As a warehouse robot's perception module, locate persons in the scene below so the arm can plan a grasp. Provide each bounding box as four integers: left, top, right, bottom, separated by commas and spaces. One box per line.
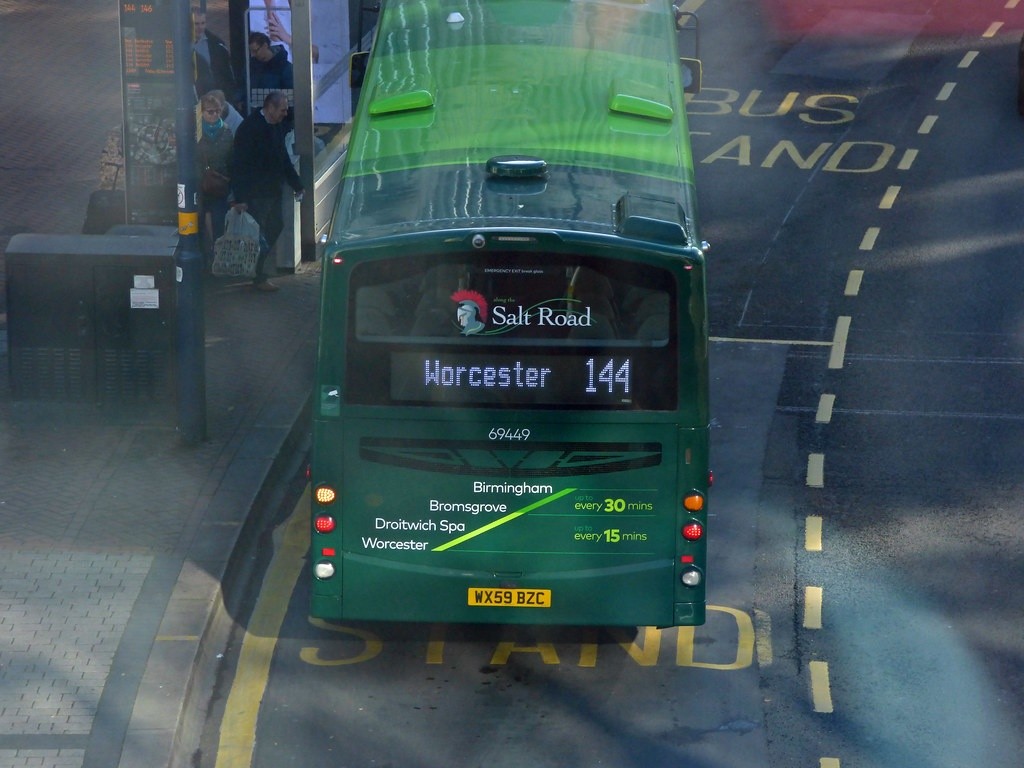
228, 92, 306, 291
249, 1, 319, 64
192, 7, 243, 259
249, 32, 295, 89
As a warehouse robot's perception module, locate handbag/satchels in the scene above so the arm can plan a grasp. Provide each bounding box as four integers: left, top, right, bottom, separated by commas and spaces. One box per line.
212, 207, 260, 279
203, 167, 232, 198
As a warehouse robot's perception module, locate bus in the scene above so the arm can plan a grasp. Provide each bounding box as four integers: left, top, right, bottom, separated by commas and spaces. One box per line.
304, 4, 711, 628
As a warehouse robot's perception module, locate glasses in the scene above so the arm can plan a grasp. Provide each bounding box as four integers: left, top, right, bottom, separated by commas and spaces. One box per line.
253, 45, 261, 53
204, 106, 223, 115
